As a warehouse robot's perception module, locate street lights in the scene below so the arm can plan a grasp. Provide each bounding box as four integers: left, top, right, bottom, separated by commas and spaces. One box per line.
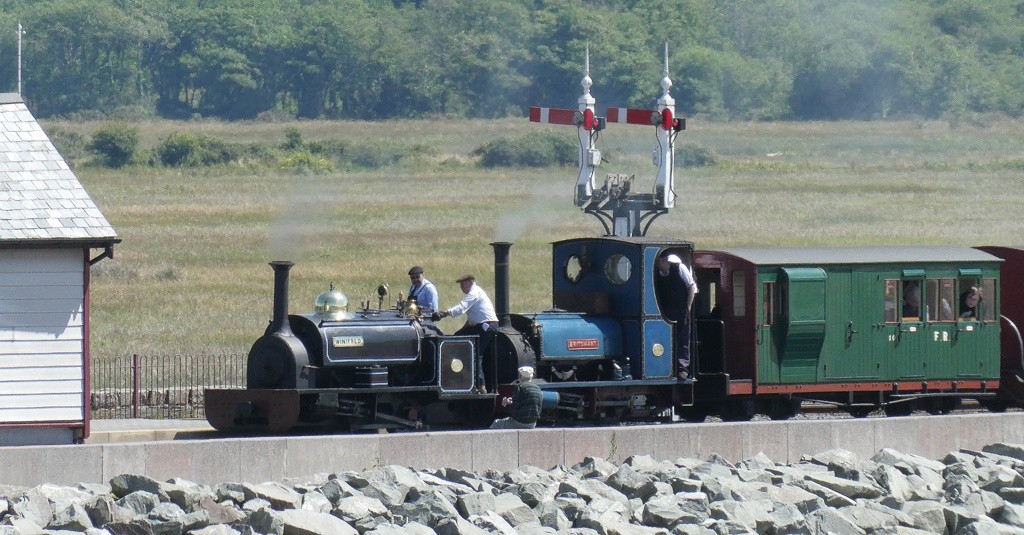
15, 20, 31, 94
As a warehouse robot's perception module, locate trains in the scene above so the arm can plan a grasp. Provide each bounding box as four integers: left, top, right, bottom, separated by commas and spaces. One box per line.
201, 40, 1024, 440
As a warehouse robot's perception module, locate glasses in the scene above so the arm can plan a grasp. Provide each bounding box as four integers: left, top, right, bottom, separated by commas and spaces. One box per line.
410, 275, 420, 279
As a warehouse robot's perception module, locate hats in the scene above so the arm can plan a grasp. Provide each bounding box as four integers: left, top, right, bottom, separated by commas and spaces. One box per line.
456, 274, 474, 282
408, 266, 423, 275
518, 366, 534, 379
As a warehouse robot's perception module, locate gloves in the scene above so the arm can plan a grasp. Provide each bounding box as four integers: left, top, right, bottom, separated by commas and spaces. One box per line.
431, 312, 445, 322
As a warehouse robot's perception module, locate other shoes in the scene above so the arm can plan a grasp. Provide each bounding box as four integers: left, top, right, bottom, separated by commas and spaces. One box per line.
678, 371, 688, 380
479, 386, 487, 394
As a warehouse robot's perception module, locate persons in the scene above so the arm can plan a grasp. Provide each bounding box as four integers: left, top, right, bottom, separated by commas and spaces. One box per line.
655, 250, 696, 379
960, 285, 983, 322
431, 275, 499, 394
407, 267, 438, 322
